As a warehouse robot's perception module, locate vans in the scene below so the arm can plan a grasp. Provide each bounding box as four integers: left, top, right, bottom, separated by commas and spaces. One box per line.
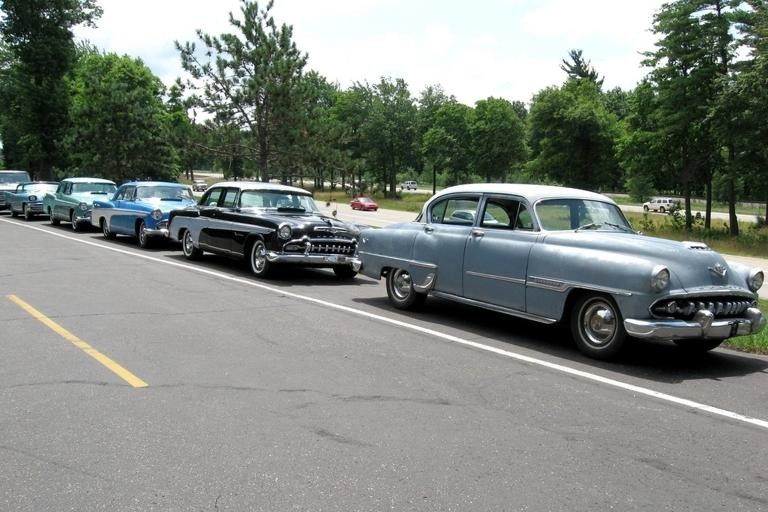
0, 170, 32, 210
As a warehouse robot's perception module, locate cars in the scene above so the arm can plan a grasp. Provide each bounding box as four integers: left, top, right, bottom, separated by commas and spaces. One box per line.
167, 181, 362, 280
350, 196, 378, 212
4, 181, 60, 221
91, 181, 197, 251
42, 177, 118, 232
351, 182, 765, 362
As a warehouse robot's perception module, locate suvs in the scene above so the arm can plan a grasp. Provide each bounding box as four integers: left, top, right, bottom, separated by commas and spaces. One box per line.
401, 180, 417, 190
192, 179, 208, 191
643, 196, 677, 213
